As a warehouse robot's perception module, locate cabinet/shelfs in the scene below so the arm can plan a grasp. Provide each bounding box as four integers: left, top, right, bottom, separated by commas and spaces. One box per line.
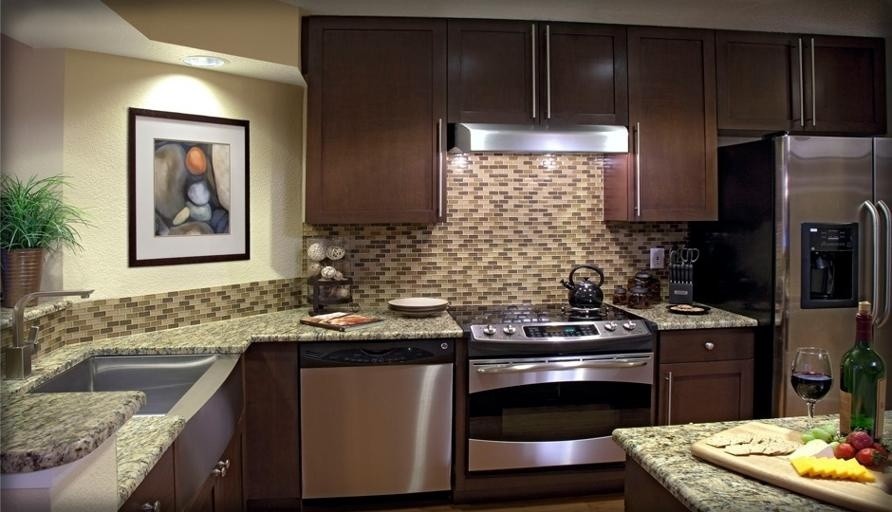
115, 442, 175, 509
714, 30, 890, 138
176, 359, 249, 512
601, 24, 716, 224
305, 12, 444, 226
659, 332, 754, 426
444, 17, 617, 123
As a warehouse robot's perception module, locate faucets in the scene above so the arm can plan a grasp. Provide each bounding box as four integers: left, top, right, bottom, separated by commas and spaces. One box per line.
5, 288, 96, 381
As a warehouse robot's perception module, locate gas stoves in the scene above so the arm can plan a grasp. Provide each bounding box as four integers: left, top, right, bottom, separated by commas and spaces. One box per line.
447, 302, 654, 357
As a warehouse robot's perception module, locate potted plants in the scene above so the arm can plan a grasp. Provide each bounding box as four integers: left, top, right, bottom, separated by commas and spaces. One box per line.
0, 170, 99, 307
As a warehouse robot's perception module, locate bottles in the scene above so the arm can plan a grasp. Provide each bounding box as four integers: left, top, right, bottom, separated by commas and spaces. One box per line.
836, 297, 884, 441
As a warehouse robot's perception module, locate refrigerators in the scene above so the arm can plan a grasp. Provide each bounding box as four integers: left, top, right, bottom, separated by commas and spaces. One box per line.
687, 135, 892, 418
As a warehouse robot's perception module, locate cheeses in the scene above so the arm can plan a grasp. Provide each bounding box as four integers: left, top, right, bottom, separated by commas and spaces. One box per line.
789, 456, 876, 482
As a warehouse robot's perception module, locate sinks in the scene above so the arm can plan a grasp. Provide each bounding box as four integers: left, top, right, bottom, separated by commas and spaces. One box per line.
10, 350, 246, 511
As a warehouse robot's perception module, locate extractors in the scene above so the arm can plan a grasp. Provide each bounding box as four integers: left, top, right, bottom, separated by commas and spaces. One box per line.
454, 122, 629, 158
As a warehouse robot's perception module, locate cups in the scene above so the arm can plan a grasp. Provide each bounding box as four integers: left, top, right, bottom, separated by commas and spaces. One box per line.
305, 237, 346, 281
612, 267, 661, 308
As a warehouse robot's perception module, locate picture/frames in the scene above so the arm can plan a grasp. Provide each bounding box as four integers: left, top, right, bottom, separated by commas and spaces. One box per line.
127, 106, 251, 267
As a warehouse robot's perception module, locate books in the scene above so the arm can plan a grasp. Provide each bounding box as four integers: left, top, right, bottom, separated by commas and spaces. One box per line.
300, 311, 385, 332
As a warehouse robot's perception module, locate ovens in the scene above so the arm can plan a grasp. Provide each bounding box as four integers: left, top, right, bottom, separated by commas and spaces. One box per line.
467, 351, 657, 477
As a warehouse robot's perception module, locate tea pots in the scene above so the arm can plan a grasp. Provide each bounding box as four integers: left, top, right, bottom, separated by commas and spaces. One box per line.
558, 264, 603, 308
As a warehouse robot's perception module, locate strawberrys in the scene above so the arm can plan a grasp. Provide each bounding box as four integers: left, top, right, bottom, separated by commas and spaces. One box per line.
835, 431, 891, 467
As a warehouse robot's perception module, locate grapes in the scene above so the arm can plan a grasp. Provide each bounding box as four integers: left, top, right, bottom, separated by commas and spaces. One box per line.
800, 425, 838, 444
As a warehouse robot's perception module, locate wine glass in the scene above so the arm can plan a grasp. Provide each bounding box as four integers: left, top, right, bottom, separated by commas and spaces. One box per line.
788, 343, 836, 432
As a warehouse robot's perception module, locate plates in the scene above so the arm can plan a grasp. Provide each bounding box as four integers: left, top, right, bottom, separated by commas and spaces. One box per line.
665, 304, 711, 314
386, 297, 448, 318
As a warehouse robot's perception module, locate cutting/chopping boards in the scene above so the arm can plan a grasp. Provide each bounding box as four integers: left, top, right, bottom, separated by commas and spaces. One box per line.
691, 419, 892, 512
